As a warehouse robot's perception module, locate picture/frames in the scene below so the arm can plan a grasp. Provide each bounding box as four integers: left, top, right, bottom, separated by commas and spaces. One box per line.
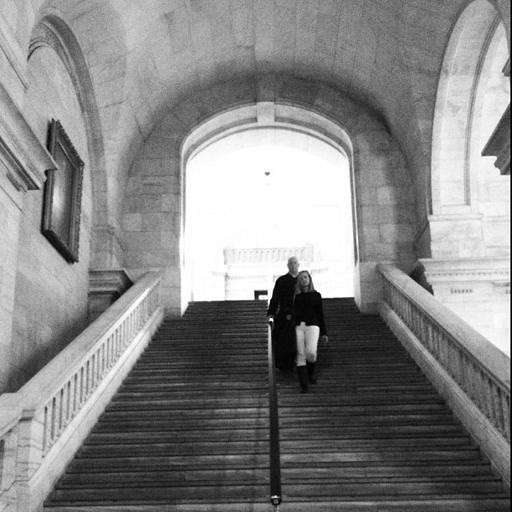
41, 118, 84, 264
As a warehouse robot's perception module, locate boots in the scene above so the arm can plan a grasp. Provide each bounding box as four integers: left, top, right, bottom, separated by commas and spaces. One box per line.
298, 365, 309, 394
307, 360, 317, 383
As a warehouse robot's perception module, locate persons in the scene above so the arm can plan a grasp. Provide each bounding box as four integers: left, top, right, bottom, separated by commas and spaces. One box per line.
266, 256, 301, 385
285, 270, 329, 394
